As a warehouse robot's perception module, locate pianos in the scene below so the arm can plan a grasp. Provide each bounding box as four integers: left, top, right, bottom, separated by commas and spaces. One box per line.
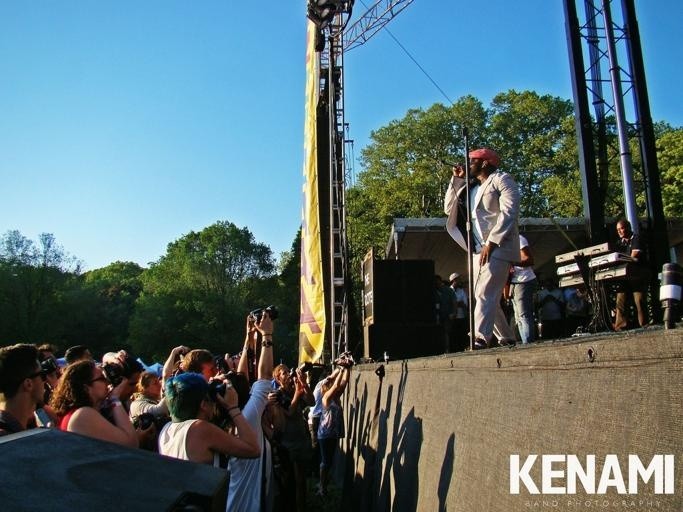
555, 242, 654, 289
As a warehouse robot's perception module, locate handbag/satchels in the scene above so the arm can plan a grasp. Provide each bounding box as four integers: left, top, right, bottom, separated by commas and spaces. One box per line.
273, 430, 300, 468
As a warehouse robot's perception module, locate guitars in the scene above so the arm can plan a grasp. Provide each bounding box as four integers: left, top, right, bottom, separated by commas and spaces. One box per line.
503, 265, 514, 301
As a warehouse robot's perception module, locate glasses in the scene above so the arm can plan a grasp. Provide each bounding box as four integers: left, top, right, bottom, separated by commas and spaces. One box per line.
33, 367, 48, 384
469, 159, 478, 165
88, 371, 108, 387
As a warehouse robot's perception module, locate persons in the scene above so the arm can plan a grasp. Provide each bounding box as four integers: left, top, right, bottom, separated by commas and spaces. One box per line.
0, 308, 356, 510
444, 148, 522, 349
431, 271, 596, 353
611, 219, 647, 330
507, 232, 539, 344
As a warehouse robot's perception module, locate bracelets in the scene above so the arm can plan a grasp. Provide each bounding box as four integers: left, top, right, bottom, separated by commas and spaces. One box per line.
483, 240, 496, 248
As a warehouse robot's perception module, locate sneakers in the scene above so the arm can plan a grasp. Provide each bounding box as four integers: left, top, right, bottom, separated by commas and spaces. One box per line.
318, 489, 329, 505
316, 483, 329, 495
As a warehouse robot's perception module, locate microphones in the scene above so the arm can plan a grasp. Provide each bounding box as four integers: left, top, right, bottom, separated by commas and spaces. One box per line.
442, 160, 465, 170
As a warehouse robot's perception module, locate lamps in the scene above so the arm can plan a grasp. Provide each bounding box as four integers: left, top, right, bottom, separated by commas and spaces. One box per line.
306, 0, 331, 30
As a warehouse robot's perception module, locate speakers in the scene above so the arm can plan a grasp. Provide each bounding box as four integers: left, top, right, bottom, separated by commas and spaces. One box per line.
370, 259, 435, 362
0, 427, 230, 512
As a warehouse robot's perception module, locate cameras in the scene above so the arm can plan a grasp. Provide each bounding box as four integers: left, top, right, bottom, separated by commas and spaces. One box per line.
40, 357, 56, 375
250, 305, 278, 322
209, 381, 225, 402
105, 360, 129, 385
289, 361, 313, 378
139, 413, 153, 430
216, 357, 229, 374
271, 389, 291, 412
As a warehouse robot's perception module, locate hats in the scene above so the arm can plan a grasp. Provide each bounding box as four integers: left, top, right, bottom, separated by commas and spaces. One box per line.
448, 272, 460, 282
467, 147, 501, 169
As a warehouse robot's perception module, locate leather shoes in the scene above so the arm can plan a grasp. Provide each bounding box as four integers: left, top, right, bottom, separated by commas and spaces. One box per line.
472, 338, 487, 350
611, 320, 627, 331
